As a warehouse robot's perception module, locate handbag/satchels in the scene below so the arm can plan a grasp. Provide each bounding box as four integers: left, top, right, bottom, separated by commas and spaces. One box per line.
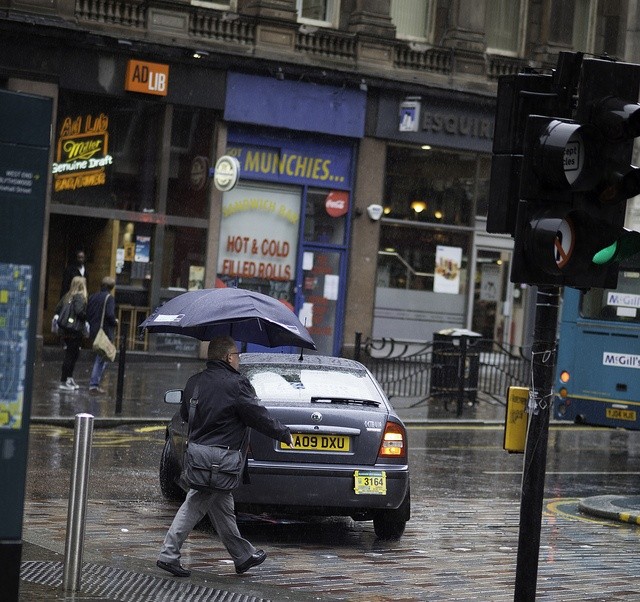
179, 370, 252, 492
92, 293, 117, 363
57, 294, 90, 340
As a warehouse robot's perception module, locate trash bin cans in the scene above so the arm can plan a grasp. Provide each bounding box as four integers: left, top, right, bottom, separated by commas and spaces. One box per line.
429, 328, 482, 419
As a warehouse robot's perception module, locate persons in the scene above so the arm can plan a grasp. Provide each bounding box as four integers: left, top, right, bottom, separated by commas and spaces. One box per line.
60, 249, 89, 303
155, 335, 296, 577
59, 274, 89, 395
87, 275, 116, 395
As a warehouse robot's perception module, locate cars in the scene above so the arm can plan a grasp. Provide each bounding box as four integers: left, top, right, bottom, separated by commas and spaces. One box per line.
159, 320, 410, 542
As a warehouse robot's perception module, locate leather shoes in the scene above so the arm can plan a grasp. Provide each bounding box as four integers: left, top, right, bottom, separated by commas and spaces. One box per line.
235, 549, 267, 574
156, 559, 191, 577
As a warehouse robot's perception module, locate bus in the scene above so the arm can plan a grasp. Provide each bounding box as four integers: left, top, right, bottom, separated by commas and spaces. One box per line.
555, 273, 640, 431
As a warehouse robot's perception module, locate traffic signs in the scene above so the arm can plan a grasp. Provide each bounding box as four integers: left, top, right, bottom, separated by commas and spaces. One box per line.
511, 113, 592, 285
486, 73, 557, 232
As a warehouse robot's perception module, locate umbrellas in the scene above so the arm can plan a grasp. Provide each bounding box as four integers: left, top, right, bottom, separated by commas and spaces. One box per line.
137, 288, 319, 353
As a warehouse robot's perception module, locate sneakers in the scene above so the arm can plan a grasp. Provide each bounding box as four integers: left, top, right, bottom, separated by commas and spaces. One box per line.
89, 385, 104, 393
59, 380, 74, 391
65, 377, 80, 390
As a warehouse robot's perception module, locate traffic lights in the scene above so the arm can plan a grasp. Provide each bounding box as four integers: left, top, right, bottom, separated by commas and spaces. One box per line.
564, 57, 640, 287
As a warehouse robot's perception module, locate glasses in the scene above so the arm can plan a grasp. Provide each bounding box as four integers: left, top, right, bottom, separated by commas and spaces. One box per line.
230, 351, 243, 357
